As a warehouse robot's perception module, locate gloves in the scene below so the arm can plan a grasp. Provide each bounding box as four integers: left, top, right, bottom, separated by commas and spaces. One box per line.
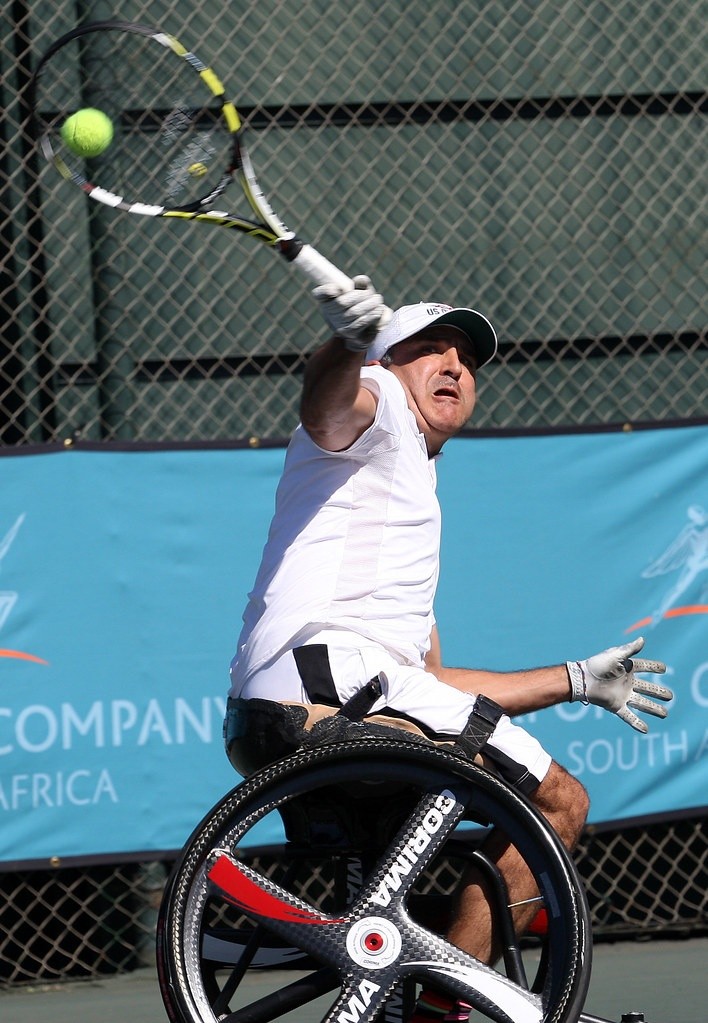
567, 637, 673, 733
312, 275, 395, 353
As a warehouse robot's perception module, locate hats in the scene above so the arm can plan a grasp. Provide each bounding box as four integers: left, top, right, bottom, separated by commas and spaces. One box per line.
365, 301, 497, 373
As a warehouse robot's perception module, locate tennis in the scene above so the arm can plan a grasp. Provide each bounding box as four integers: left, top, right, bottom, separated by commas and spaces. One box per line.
61, 108, 118, 157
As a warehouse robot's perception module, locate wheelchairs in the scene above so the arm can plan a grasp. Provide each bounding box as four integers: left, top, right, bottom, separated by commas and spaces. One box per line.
155, 673, 648, 1023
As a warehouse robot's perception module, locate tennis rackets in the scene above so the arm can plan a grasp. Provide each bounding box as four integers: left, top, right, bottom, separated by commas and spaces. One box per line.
30, 19, 395, 333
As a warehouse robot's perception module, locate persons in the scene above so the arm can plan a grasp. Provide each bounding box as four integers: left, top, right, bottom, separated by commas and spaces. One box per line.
225, 275, 673, 1021
